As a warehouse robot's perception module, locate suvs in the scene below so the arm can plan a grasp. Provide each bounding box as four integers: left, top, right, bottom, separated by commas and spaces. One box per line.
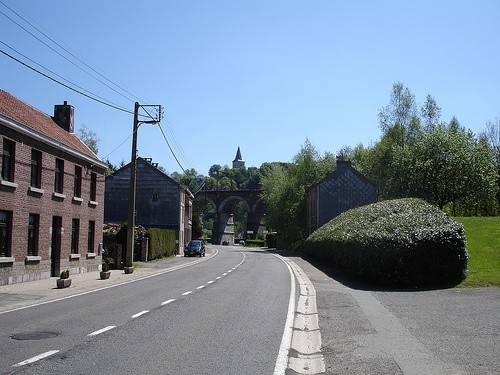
184, 240, 205, 258
